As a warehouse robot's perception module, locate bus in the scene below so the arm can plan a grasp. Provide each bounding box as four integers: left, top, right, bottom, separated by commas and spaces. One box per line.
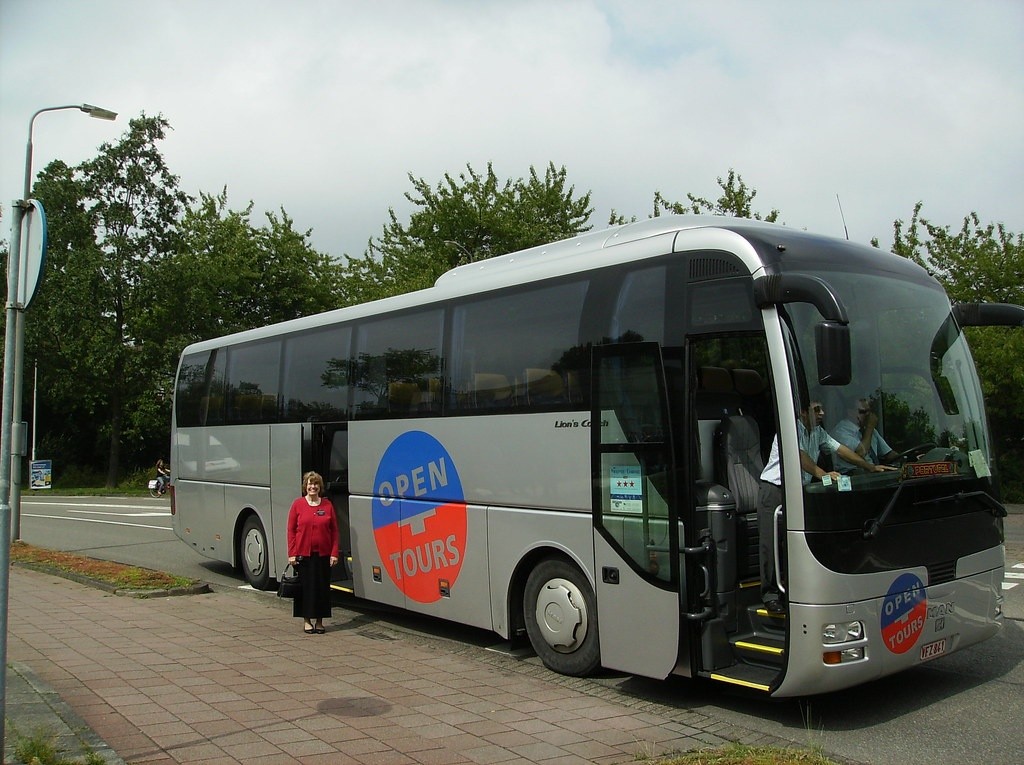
168, 212, 1024, 701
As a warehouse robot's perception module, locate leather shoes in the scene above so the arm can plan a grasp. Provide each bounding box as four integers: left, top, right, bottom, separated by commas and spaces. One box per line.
304, 624, 315, 634
760, 596, 784, 613
315, 624, 325, 634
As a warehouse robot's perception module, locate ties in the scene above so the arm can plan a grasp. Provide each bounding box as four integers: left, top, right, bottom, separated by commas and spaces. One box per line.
859, 427, 879, 464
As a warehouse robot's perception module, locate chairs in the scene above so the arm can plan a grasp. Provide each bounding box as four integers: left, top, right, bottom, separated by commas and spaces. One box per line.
386, 365, 586, 413
698, 364, 729, 407
715, 413, 770, 518
200, 389, 316, 422
733, 362, 779, 402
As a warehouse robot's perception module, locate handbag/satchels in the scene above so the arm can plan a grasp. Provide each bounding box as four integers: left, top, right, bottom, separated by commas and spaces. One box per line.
276, 562, 305, 598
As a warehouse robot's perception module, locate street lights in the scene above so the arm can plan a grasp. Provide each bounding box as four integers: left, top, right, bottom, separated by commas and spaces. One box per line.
0, 103, 118, 765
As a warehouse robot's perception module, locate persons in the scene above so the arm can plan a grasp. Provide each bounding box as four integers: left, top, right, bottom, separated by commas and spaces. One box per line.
759, 393, 897, 613
831, 396, 924, 476
157, 459, 170, 495
287, 472, 339, 634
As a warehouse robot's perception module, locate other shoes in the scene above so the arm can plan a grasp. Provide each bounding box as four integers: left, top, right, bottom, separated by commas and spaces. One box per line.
157, 491, 161, 495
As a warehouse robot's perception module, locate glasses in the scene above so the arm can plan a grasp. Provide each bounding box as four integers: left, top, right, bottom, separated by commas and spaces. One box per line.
859, 409, 867, 414
808, 405, 820, 412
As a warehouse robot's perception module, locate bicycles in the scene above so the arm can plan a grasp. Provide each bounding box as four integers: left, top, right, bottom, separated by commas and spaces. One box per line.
149, 471, 170, 498
